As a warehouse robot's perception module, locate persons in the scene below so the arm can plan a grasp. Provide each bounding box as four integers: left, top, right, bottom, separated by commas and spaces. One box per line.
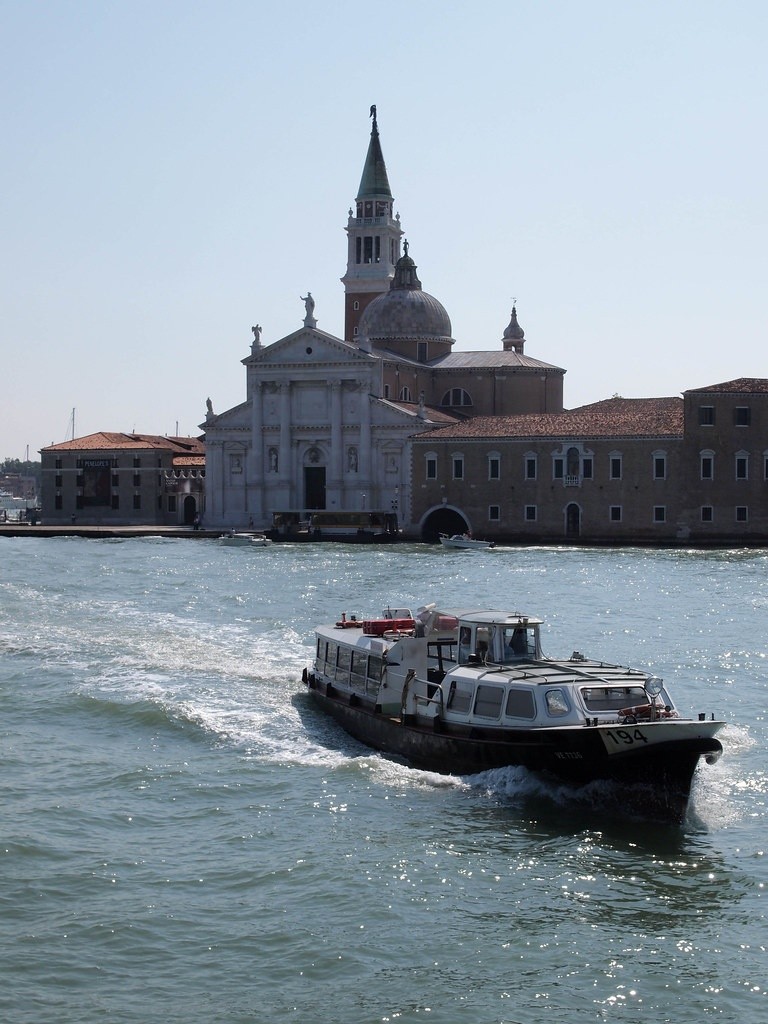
464, 530, 471, 541
300, 292, 315, 320
270, 451, 277, 471
193, 512, 200, 530
71, 514, 75, 525
403, 239, 409, 255
307, 518, 311, 533
417, 391, 425, 409
248, 517, 254, 530
252, 324, 262, 342
368, 513, 374, 528
349, 451, 357, 469
228, 528, 235, 538
206, 397, 212, 411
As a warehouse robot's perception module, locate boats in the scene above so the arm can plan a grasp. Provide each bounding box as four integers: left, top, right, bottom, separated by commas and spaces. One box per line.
438, 532, 495, 549
301, 602, 728, 827
268, 507, 399, 544
217, 529, 273, 547
